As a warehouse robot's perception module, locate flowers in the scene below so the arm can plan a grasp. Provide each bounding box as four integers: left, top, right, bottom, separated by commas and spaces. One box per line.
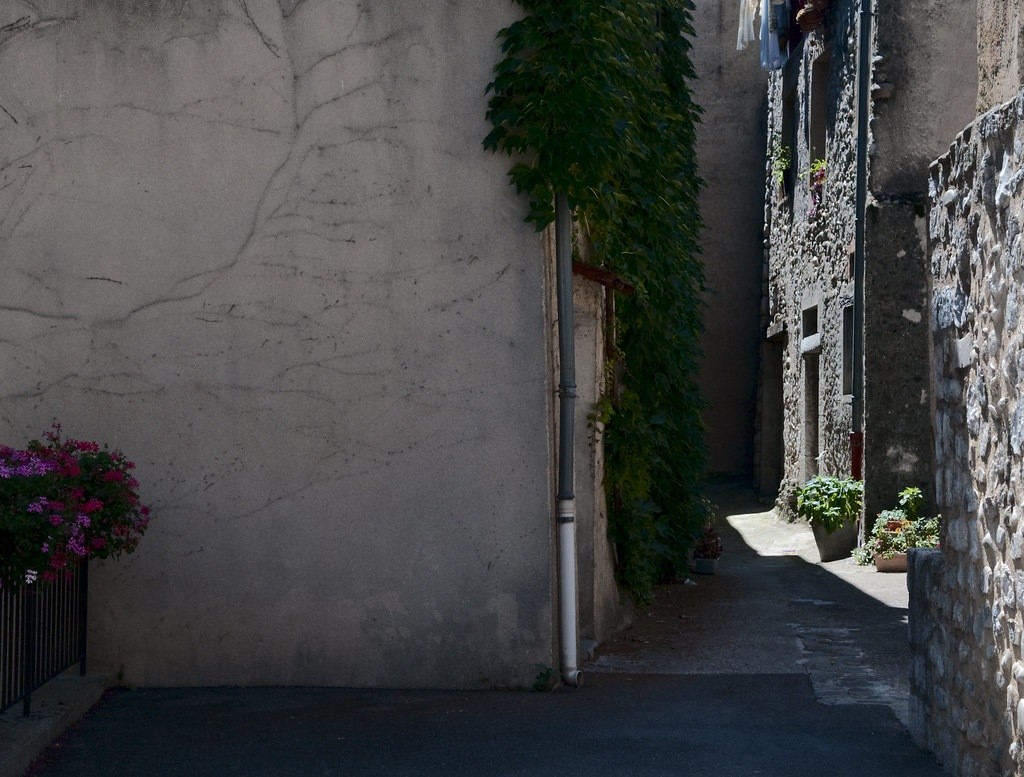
0, 420, 155, 584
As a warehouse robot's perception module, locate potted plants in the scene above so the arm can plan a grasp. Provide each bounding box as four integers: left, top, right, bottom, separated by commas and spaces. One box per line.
800, 159, 826, 203
866, 486, 942, 572
692, 529, 723, 575
796, 474, 864, 562
